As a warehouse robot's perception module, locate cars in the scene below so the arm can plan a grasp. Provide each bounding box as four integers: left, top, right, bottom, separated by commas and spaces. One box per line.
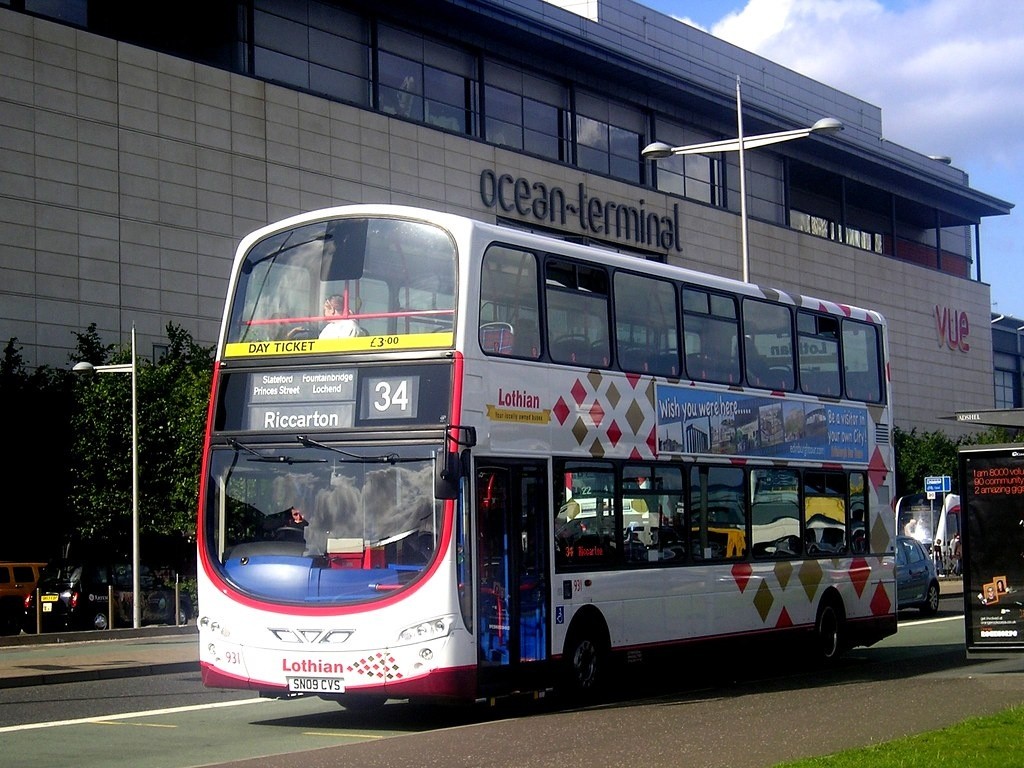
21, 554, 192, 631
896, 535, 940, 616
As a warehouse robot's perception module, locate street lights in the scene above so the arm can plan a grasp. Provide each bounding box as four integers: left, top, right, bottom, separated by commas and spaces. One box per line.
70, 321, 144, 628
636, 73, 844, 282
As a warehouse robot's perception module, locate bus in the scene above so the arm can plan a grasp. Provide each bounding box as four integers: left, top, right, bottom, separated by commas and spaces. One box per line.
194, 202, 900, 719
895, 492, 960, 576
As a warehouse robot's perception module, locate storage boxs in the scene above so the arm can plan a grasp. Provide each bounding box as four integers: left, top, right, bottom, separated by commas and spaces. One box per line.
327, 538, 386, 570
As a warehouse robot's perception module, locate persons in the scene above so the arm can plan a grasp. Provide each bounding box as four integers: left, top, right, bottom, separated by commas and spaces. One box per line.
932, 533, 962, 577
313, 295, 368, 337
280, 505, 309, 543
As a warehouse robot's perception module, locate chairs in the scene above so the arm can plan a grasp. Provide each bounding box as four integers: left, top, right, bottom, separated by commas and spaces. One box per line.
479, 321, 873, 400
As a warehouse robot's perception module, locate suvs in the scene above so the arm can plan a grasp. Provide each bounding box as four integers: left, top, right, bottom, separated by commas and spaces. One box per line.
0, 561, 50, 628
